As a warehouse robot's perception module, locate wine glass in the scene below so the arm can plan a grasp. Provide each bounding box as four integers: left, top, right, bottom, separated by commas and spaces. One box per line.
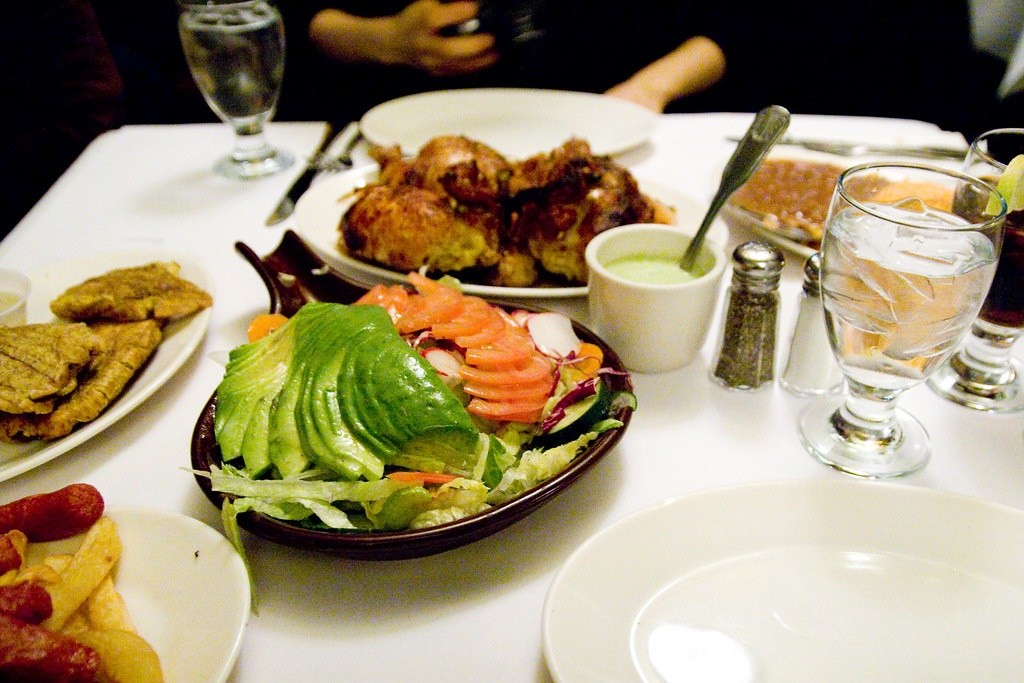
797, 162, 1007, 477
925, 127, 1024, 413
177, 2, 296, 180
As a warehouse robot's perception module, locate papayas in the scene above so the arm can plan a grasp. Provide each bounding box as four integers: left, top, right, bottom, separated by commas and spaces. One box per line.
216, 303, 479, 480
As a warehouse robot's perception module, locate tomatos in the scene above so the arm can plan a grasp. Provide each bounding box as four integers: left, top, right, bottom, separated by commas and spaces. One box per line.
352, 272, 552, 423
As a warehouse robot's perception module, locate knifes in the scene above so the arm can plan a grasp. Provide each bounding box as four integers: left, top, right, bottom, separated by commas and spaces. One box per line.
266, 121, 350, 225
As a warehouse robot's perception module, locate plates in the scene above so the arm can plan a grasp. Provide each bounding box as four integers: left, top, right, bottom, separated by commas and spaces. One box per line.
191, 230, 636, 560
0, 249, 213, 482
541, 478, 1024, 683
360, 88, 660, 156
714, 147, 854, 241
289, 158, 701, 297
27, 505, 252, 683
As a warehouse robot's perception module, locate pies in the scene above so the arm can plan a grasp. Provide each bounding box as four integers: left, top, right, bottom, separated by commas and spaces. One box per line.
0, 261, 212, 442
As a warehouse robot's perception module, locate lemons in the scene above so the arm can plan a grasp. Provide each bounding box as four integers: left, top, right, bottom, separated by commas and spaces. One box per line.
985, 154, 1024, 215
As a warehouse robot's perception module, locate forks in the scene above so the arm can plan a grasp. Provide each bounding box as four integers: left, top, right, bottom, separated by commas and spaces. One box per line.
303, 130, 362, 172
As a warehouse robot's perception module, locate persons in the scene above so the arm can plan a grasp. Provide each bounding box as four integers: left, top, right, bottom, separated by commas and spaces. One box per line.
284, 0, 776, 132
0, 0, 125, 225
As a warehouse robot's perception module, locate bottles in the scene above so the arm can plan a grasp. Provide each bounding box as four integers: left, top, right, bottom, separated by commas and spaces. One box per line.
710, 240, 786, 392
778, 252, 845, 399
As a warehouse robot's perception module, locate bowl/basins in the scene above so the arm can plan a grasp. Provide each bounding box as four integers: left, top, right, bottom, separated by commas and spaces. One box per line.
0, 268, 32, 328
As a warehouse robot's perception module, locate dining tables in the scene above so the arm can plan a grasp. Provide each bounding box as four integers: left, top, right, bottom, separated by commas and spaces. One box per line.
0, 116, 1024, 683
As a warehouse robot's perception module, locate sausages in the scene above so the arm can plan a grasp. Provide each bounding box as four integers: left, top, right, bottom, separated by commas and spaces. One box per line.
0, 483, 116, 683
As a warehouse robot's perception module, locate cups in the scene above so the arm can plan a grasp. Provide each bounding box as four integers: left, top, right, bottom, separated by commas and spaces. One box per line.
585, 223, 725, 373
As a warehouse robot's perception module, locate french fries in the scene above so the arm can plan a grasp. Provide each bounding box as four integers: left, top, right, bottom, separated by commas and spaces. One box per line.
0, 515, 163, 683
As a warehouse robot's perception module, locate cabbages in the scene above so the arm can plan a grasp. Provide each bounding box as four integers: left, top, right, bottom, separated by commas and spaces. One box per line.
181, 423, 596, 615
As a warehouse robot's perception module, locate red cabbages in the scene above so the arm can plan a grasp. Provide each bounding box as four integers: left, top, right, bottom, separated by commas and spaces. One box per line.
402, 304, 630, 433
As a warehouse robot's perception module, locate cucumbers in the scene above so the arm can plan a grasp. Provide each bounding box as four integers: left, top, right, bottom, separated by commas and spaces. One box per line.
538, 381, 610, 444
